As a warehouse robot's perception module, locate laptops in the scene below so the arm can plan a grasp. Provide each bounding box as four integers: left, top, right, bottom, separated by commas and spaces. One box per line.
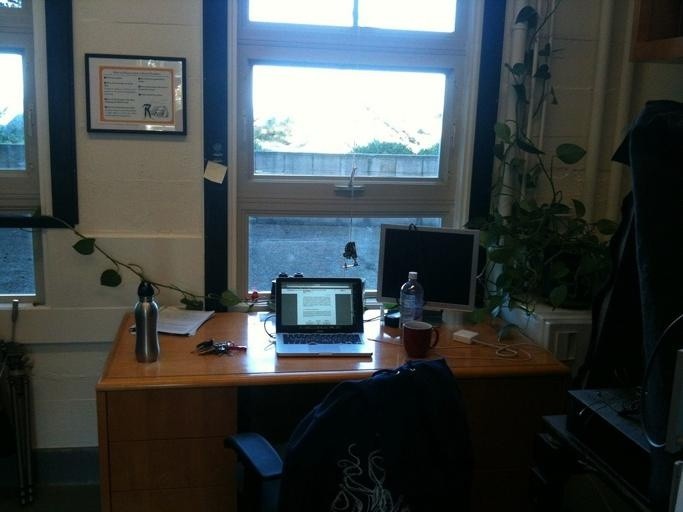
275, 277, 372, 357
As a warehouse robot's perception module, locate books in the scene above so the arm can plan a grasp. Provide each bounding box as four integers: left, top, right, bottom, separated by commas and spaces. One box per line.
128, 305, 215, 337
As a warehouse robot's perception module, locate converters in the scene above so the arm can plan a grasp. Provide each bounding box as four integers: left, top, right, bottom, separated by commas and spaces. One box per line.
453, 330, 480, 346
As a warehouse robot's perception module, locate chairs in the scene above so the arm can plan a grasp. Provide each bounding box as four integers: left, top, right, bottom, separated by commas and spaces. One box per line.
223, 357, 474, 511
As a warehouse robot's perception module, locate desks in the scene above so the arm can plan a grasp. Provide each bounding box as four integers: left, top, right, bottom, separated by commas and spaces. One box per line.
500, 299, 592, 362
93, 308, 567, 512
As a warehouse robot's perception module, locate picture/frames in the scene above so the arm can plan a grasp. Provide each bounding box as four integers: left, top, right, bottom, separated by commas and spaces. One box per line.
83, 53, 187, 135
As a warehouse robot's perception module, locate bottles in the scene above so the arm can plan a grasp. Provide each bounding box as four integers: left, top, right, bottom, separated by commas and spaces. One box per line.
133, 280, 160, 365
398, 271, 424, 339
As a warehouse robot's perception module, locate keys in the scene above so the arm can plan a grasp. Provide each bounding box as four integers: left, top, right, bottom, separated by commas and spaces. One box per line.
189, 339, 247, 360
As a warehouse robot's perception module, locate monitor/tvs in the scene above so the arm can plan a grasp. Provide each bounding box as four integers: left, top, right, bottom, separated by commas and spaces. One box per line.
376, 223, 480, 328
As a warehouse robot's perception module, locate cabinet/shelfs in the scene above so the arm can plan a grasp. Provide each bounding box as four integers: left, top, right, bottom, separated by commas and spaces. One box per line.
628, 0, 683, 64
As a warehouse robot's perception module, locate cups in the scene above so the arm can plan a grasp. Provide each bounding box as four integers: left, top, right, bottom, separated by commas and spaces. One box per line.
402, 321, 439, 358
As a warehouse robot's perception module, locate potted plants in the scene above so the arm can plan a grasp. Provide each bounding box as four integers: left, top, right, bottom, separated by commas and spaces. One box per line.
32, 0, 617, 339
